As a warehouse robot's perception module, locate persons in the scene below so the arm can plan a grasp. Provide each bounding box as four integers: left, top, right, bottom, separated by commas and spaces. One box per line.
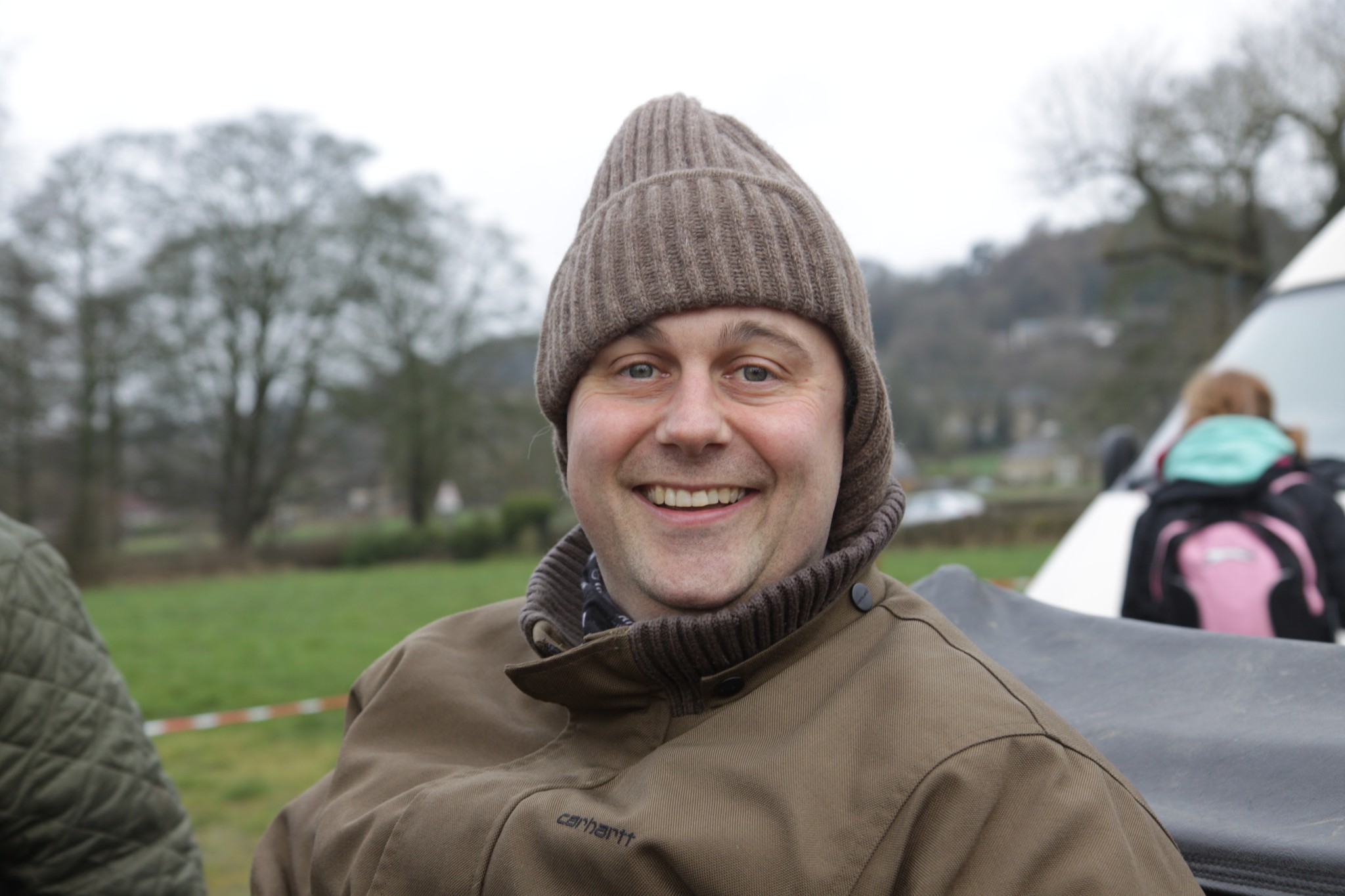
248, 95, 1203, 896
1119, 369, 1345, 647
1, 513, 209, 896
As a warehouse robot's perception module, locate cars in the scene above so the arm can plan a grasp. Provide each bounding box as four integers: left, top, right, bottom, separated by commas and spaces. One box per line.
1025, 209, 1345, 636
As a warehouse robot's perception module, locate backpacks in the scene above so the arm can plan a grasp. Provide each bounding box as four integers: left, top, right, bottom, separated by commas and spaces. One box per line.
1146, 471, 1337, 644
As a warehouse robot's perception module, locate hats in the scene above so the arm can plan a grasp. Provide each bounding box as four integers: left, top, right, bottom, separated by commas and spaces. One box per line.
535, 95, 896, 551
